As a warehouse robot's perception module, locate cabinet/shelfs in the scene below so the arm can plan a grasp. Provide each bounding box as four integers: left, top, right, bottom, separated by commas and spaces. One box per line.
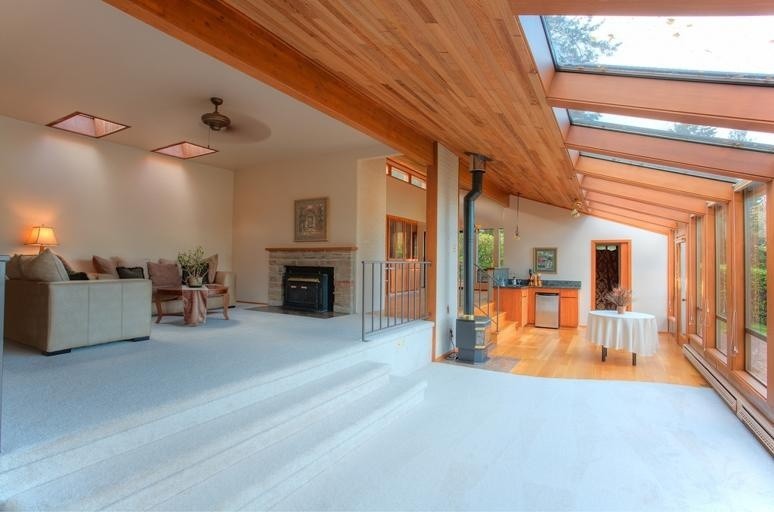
560, 288, 580, 328
494, 288, 535, 329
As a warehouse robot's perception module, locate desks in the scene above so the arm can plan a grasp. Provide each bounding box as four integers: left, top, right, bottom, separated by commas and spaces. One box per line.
588, 310, 659, 365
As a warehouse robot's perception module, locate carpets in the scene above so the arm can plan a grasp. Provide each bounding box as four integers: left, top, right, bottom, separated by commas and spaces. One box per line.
442, 352, 520, 373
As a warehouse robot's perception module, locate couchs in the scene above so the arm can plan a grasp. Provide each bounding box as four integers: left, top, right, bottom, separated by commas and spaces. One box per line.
5, 248, 235, 356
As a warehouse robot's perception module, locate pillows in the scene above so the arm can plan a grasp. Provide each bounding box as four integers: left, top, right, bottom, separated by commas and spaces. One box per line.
6, 247, 219, 302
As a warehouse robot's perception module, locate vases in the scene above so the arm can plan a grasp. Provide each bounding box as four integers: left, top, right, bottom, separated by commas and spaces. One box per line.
617, 305, 628, 315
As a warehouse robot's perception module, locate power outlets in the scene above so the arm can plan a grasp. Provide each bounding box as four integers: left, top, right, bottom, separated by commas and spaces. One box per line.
448, 328, 453, 340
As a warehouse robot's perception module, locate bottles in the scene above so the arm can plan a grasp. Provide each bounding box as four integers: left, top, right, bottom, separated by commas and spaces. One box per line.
512, 277, 516, 285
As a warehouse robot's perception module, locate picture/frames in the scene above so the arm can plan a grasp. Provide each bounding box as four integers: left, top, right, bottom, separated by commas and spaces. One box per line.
532, 248, 557, 275
293, 197, 328, 242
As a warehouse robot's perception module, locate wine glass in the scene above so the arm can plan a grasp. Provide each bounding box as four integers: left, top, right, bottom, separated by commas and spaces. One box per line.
518, 279, 522, 286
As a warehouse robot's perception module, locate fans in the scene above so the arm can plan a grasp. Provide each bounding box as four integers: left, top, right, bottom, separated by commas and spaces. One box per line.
176, 97, 271, 144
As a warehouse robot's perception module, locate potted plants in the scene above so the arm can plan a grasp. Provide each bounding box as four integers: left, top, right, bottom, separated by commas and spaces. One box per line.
605, 286, 632, 314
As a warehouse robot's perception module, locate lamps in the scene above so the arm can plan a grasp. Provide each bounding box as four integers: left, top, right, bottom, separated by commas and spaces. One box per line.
475, 201, 481, 231
24, 224, 59, 254
512, 193, 522, 239
571, 201, 582, 218
